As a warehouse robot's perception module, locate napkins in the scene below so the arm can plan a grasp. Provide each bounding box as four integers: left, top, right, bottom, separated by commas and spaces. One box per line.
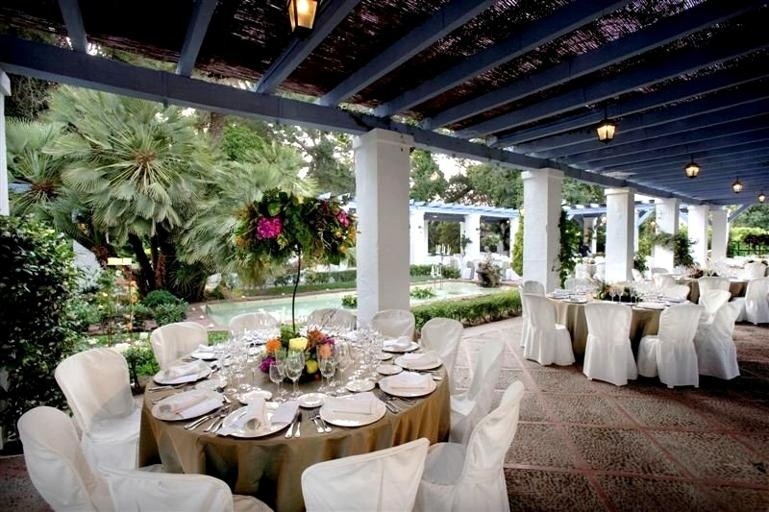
162, 358, 208, 378
158, 388, 209, 414
244, 392, 268, 432
402, 351, 438, 367
389, 373, 432, 389
386, 336, 413, 350
198, 344, 214, 351
320, 396, 378, 416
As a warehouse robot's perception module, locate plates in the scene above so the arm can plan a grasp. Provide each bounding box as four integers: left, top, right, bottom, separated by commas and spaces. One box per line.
151, 366, 209, 387
395, 355, 448, 371
147, 386, 224, 423
380, 339, 423, 353
189, 346, 217, 359
316, 392, 385, 428
222, 405, 291, 439
380, 374, 438, 400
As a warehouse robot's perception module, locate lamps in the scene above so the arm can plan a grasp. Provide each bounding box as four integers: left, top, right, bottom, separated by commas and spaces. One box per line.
287, 0, 318, 36
592, 97, 617, 144
758, 189, 767, 203
730, 176, 743, 193
683, 153, 701, 179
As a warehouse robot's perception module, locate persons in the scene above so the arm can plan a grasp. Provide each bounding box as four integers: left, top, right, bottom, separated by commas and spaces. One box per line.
577, 240, 591, 256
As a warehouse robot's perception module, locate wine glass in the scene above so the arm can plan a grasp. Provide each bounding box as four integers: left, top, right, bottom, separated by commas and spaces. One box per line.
679, 261, 733, 279
570, 276, 648, 305
214, 316, 384, 398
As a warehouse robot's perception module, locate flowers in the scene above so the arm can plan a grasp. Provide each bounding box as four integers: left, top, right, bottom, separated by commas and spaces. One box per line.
260, 324, 337, 378
219, 183, 363, 335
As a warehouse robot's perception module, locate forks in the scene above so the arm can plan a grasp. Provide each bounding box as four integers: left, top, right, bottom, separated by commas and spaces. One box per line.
307, 406, 333, 434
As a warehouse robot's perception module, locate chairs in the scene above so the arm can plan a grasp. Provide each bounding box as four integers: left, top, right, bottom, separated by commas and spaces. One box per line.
305, 308, 356, 331
54, 346, 144, 474
229, 312, 280, 338
412, 380, 527, 511
448, 339, 505, 443
369, 308, 416, 343
300, 438, 430, 512
96, 463, 273, 510
419, 317, 464, 396
518, 251, 769, 391
150, 321, 209, 371
18, 405, 168, 511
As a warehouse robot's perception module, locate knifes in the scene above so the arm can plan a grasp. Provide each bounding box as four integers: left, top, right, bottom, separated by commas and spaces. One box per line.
295, 411, 302, 436
